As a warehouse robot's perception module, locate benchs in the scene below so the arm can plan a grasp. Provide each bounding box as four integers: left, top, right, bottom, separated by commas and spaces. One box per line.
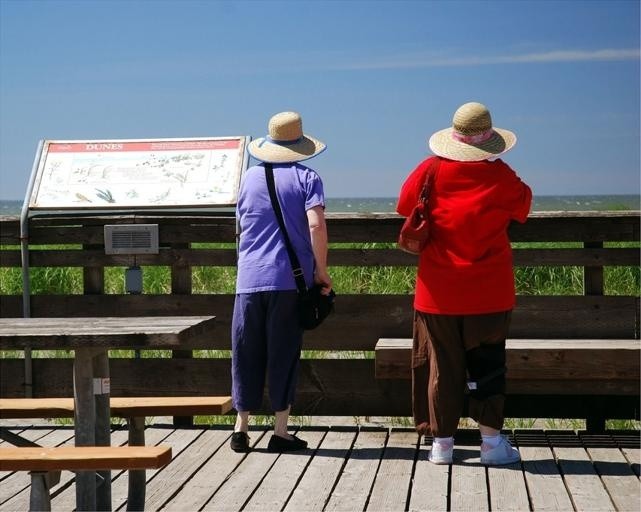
372, 335, 640, 438
0, 395, 234, 511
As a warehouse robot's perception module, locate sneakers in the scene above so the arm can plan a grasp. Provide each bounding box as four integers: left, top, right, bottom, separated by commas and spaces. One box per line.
428, 436, 455, 465
229, 431, 250, 452
266, 434, 308, 453
480, 436, 522, 466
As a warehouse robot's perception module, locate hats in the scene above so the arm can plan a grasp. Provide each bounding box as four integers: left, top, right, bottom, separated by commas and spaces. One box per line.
428, 102, 518, 162
245, 111, 328, 165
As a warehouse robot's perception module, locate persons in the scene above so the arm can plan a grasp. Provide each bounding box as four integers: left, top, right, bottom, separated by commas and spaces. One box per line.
395, 102, 532, 465
231, 111, 331, 452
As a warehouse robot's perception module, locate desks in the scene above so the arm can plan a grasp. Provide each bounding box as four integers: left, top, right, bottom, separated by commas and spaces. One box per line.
1, 313, 215, 511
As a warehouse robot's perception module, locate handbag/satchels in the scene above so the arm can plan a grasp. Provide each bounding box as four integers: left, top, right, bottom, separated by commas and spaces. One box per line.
290, 279, 337, 331
396, 156, 442, 255
